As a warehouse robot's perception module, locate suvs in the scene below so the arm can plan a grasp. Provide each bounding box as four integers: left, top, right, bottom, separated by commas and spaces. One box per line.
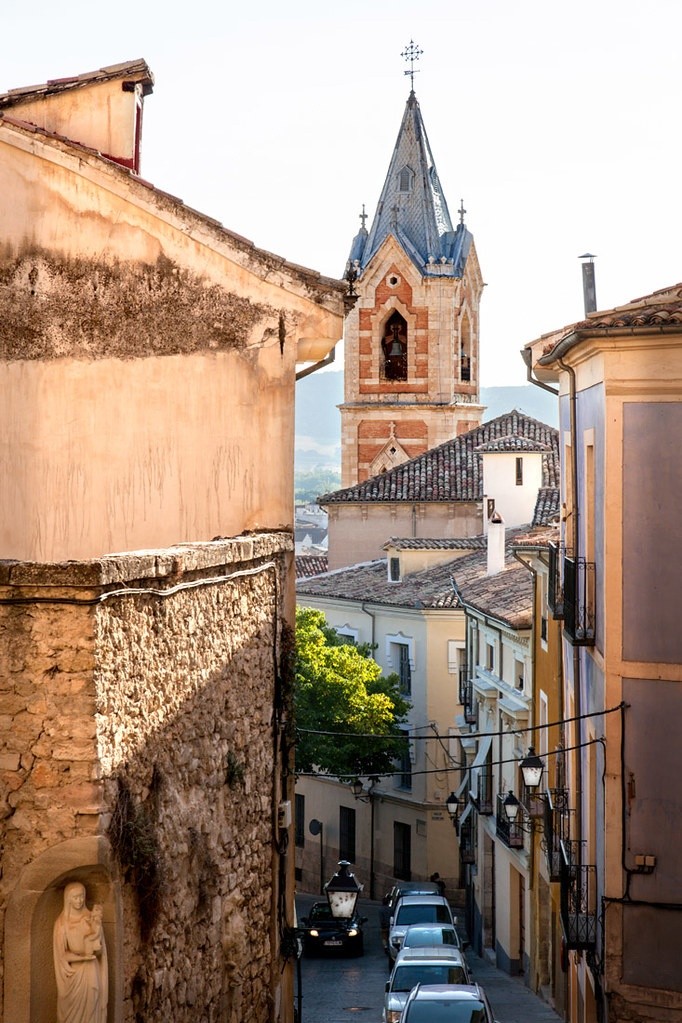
398, 980, 501, 1023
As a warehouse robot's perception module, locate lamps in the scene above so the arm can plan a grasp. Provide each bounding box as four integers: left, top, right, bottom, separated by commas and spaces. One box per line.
349, 779, 370, 803
281, 859, 364, 957
516, 746, 567, 809
445, 792, 467, 820
502, 790, 531, 833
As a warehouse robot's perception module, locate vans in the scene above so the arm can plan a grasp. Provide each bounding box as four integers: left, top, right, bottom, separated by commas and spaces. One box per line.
381, 880, 443, 912
382, 894, 459, 973
381, 943, 473, 1023
398, 922, 469, 953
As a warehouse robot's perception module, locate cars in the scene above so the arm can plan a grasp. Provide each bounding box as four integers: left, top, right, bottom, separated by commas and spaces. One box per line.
300, 900, 369, 960
